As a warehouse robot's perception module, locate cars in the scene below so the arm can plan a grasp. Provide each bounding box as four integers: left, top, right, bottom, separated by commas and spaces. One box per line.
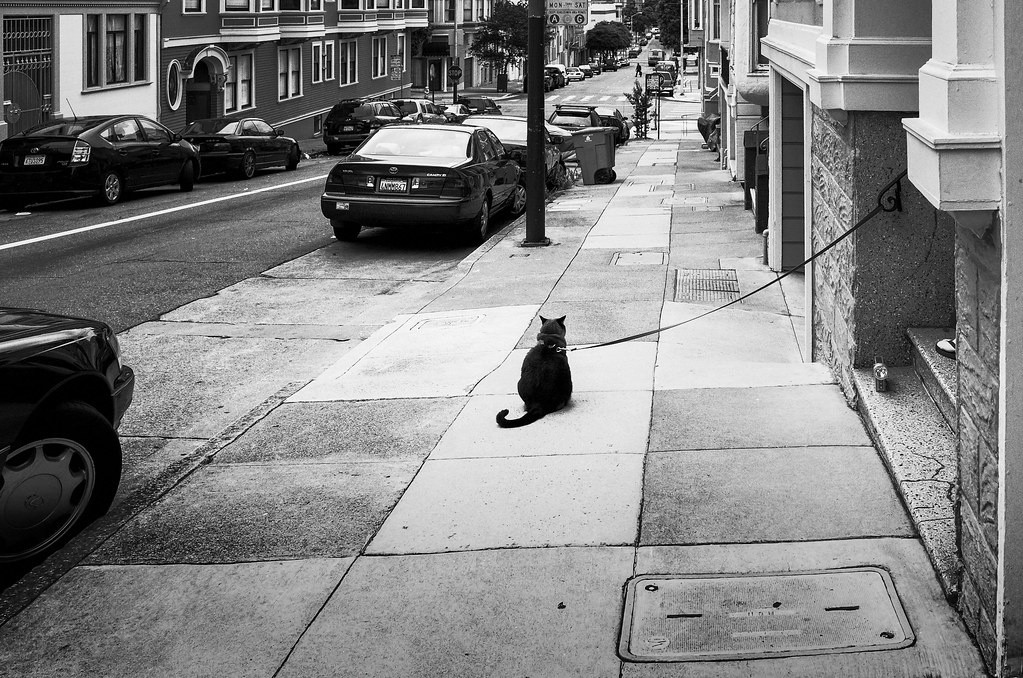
176, 115, 303, 179
0, 114, 203, 212
0, 303, 135, 585
436, 103, 472, 125
593, 105, 631, 145
321, 121, 526, 242
460, 114, 567, 198
524, 27, 679, 98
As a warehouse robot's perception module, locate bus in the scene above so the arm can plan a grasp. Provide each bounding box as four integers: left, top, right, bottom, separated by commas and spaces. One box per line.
648, 49, 666, 66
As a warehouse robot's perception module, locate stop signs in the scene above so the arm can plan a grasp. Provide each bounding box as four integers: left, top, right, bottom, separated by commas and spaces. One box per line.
447, 66, 462, 81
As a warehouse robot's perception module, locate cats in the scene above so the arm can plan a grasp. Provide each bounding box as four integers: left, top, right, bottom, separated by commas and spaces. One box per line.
496, 315, 573, 429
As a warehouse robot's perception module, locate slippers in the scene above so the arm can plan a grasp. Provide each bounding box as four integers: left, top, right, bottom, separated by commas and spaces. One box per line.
935, 339, 955, 359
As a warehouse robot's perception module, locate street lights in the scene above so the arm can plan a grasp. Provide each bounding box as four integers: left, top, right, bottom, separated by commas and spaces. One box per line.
631, 12, 642, 47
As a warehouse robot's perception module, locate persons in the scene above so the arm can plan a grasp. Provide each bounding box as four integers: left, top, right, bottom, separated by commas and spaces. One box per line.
635, 63, 642, 77
695, 57, 698, 66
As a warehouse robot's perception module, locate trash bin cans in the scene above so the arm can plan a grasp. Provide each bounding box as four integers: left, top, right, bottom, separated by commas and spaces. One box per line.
572, 127, 619, 185
497, 74, 508, 92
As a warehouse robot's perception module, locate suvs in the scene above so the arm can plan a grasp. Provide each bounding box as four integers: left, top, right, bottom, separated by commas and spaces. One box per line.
548, 102, 603, 163
386, 98, 449, 124
456, 95, 502, 116
323, 97, 409, 156
